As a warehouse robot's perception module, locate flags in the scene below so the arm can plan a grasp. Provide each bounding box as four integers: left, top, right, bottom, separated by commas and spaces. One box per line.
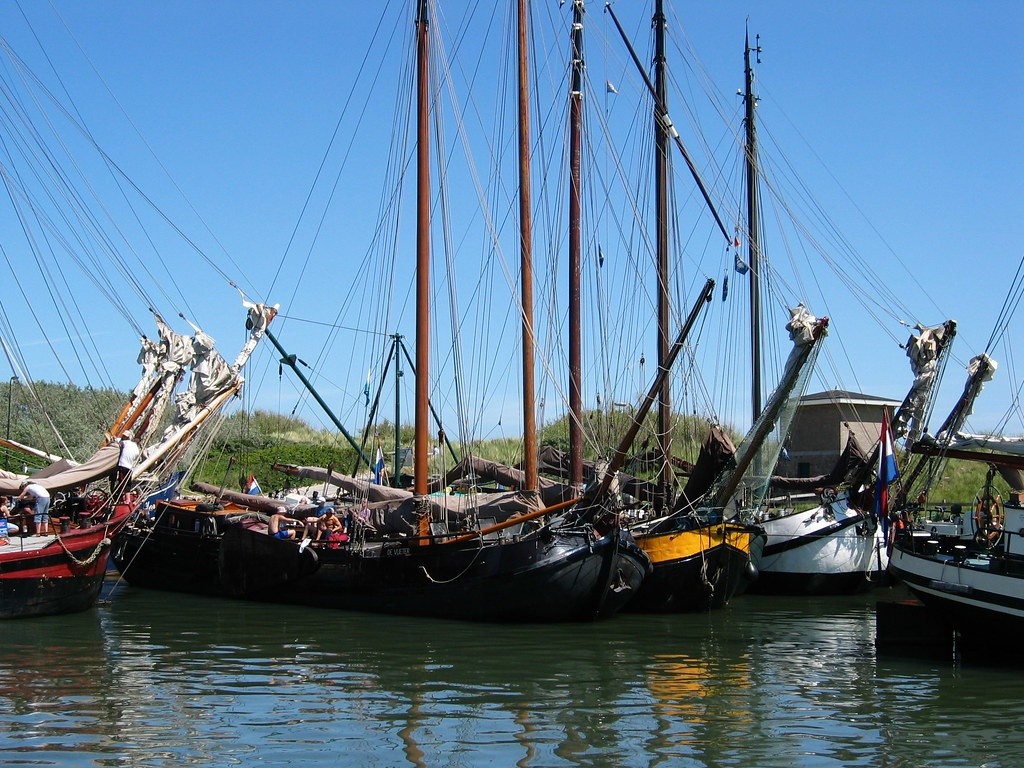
244, 476, 261, 495
374, 438, 386, 484
876, 407, 900, 547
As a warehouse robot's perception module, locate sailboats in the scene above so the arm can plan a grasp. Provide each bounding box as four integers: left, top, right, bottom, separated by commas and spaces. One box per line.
1, 0, 1024, 649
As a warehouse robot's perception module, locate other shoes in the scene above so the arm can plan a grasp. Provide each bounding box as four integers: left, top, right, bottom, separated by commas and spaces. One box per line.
31, 532, 49, 537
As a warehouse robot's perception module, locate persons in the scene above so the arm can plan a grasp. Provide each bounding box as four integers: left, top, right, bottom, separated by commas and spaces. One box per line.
12, 481, 51, 537
0, 496, 19, 534
118, 438, 140, 504
305, 508, 342, 546
313, 498, 336, 516
104, 430, 132, 497
268, 506, 304, 540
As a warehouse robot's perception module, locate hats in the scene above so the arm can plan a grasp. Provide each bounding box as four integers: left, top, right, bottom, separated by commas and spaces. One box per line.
317, 497, 326, 504
277, 506, 287, 513
18, 480, 27, 489
